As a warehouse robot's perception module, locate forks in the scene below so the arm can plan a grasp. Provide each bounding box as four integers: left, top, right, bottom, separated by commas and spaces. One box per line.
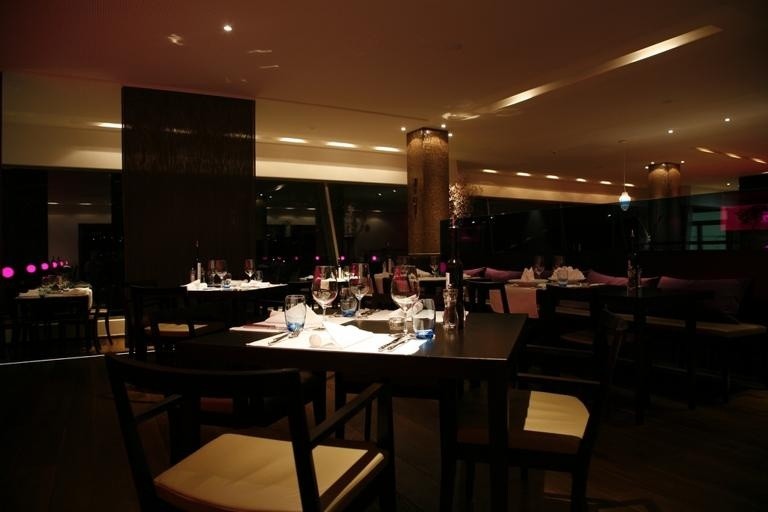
387, 339, 411, 350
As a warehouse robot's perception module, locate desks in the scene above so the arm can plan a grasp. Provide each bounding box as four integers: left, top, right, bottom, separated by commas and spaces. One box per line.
170, 301, 530, 511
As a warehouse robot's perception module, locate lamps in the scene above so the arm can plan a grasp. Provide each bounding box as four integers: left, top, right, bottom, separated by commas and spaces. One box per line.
616, 136, 632, 212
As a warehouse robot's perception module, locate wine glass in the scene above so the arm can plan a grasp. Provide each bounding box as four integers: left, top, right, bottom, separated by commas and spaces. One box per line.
390, 266, 419, 334
533, 255, 543, 279
312, 266, 338, 328
40, 275, 69, 294
244, 259, 254, 282
429, 256, 438, 277
206, 260, 231, 287
348, 262, 369, 318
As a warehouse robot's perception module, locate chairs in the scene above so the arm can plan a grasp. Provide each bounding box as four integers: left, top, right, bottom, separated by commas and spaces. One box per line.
458, 312, 645, 510
247, 257, 766, 386
2, 273, 113, 358
121, 259, 288, 351
104, 353, 396, 512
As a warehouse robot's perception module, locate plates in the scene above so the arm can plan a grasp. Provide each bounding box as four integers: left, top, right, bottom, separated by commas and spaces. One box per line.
506, 279, 548, 286
547, 277, 586, 283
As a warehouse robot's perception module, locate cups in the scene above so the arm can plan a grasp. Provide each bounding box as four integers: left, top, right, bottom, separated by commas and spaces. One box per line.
556, 270, 567, 287
284, 295, 305, 337
412, 299, 435, 339
554, 256, 567, 269
339, 286, 357, 318
389, 317, 407, 338
442, 285, 457, 329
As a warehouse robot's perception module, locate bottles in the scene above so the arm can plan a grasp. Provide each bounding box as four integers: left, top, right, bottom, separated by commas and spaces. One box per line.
322, 266, 368, 278
626, 229, 640, 298
381, 257, 395, 274
255, 270, 262, 280
188, 239, 205, 283
445, 228, 463, 329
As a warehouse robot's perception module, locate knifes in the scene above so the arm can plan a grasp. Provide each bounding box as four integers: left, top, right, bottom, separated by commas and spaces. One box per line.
267, 332, 288, 347
242, 323, 287, 330
378, 337, 401, 352
